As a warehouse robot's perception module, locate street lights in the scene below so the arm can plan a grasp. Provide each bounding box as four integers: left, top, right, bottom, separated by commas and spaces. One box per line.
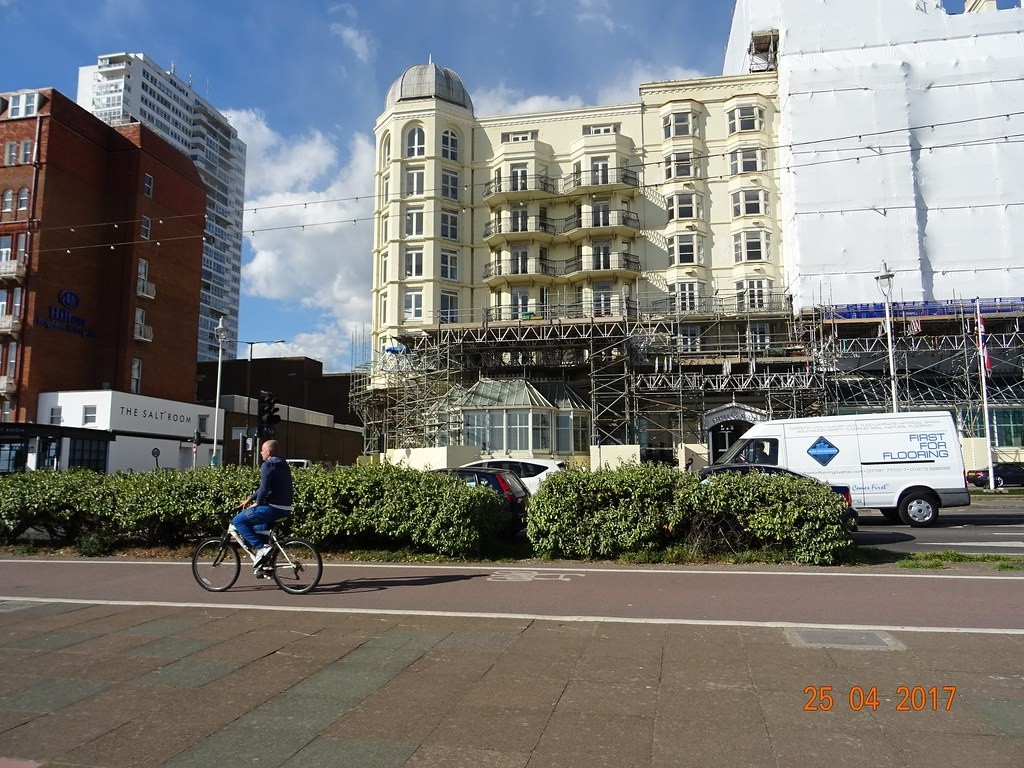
875, 258, 900, 413
219, 337, 286, 463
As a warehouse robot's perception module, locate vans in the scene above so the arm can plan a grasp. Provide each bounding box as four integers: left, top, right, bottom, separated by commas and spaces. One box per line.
702, 411, 971, 528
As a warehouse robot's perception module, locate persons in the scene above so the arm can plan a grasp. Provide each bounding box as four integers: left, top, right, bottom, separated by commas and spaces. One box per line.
745, 443, 769, 463
232, 440, 294, 578
673, 458, 693, 474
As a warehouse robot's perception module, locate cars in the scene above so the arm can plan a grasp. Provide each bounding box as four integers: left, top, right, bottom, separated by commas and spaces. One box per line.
967, 461, 1024, 488
284, 461, 314, 474
419, 466, 531, 536
689, 463, 860, 542
456, 456, 567, 500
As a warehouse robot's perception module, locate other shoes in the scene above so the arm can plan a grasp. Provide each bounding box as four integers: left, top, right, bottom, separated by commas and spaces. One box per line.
253, 543, 274, 567
254, 566, 274, 579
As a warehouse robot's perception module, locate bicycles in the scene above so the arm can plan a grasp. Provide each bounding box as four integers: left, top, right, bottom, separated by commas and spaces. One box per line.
192, 500, 323, 594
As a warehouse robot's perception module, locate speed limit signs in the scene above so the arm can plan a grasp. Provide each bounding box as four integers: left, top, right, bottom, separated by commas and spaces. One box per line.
152, 448, 161, 459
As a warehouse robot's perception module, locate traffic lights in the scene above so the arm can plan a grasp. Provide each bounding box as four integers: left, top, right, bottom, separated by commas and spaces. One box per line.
255, 390, 282, 440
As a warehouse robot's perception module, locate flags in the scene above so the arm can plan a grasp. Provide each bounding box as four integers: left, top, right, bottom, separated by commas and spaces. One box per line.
975, 304, 992, 381
